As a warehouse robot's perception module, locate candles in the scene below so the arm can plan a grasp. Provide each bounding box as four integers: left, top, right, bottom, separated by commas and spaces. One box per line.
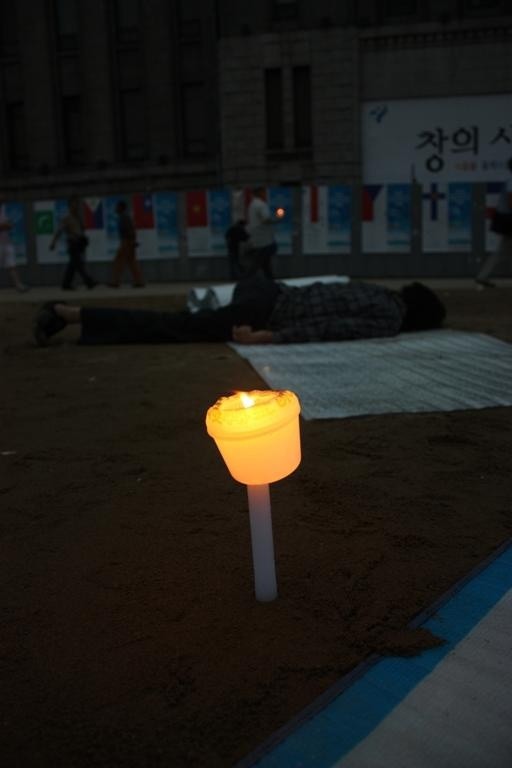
204, 386, 305, 487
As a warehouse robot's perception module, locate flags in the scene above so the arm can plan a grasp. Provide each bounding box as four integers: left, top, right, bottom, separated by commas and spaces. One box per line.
184, 186, 353, 257
8, 192, 184, 268
359, 184, 472, 253
484, 182, 501, 249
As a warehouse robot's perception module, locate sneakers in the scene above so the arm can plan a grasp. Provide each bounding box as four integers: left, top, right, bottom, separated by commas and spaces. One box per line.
33, 299, 66, 347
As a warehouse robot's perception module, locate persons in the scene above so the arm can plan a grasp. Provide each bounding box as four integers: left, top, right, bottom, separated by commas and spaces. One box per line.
1, 201, 24, 293
246, 186, 280, 276
474, 180, 512, 286
50, 195, 99, 293
105, 202, 148, 288
28, 277, 447, 344
